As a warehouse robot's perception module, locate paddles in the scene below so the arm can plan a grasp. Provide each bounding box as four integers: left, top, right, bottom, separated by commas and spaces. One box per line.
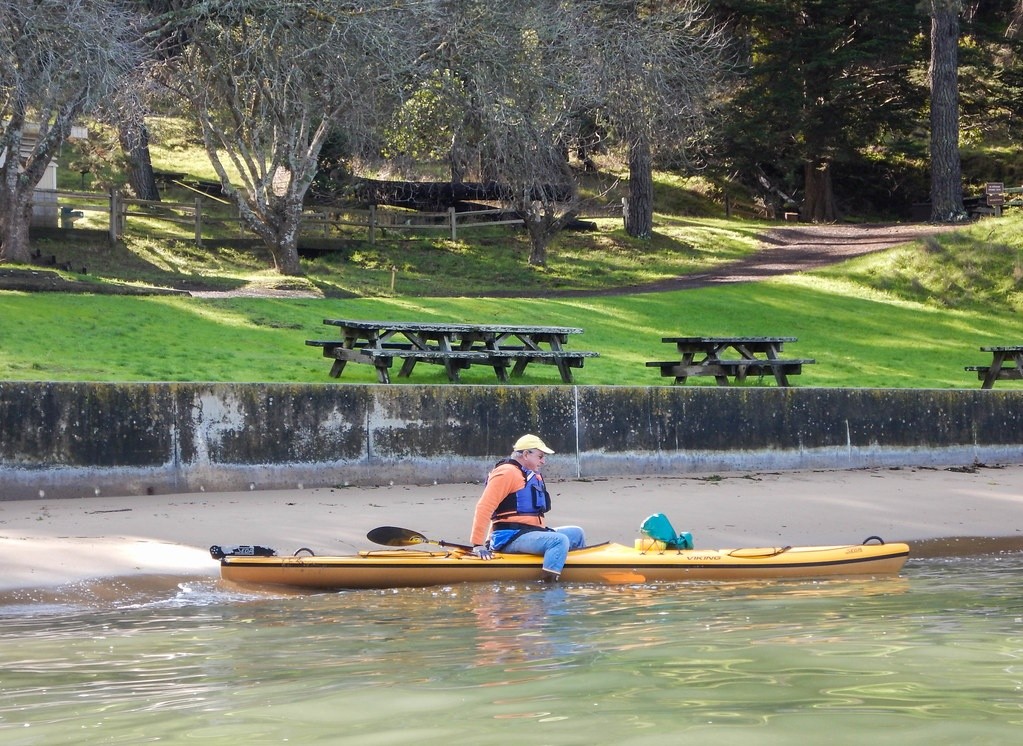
367, 526, 473, 551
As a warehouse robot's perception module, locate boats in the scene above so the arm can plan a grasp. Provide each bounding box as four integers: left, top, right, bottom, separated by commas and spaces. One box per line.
208, 535, 912, 594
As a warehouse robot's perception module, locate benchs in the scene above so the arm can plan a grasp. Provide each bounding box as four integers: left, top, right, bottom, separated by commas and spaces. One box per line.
965, 367, 1023, 380
646, 359, 815, 387
306, 341, 600, 383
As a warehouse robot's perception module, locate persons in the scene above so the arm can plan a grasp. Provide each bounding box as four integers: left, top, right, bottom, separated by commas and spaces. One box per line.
470, 433, 586, 584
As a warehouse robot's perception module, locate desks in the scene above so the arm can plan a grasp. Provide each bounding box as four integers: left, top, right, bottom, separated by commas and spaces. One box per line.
323, 320, 583, 384
981, 346, 1023, 389
662, 337, 798, 387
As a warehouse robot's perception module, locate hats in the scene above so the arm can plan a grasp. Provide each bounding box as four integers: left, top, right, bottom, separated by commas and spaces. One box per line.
513, 434, 555, 453
